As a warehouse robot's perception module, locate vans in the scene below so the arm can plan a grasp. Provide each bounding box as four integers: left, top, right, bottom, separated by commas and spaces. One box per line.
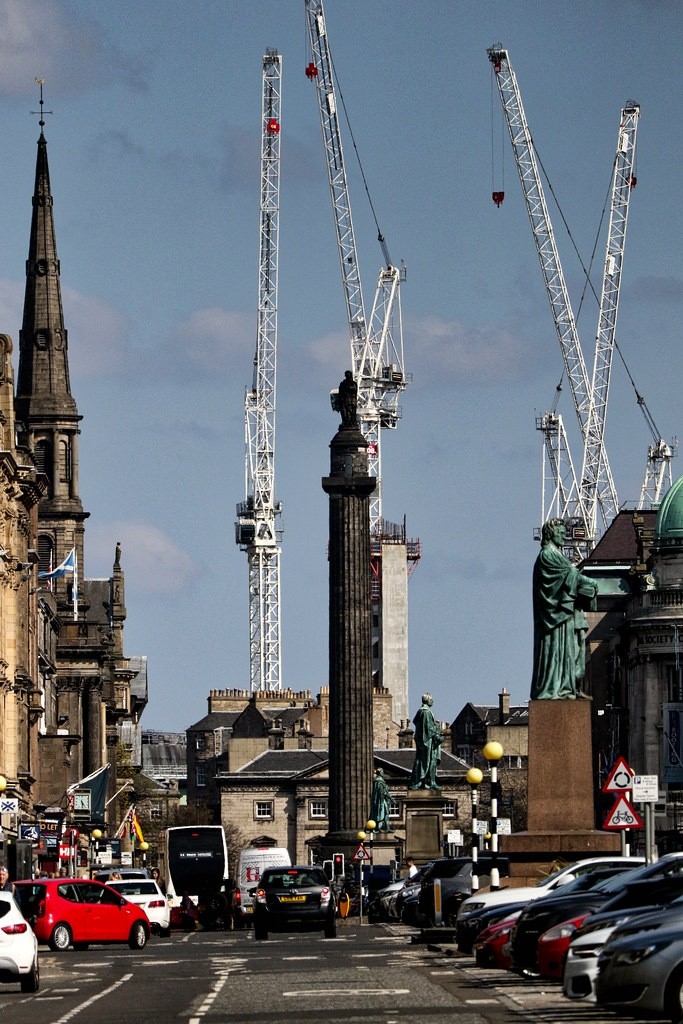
230, 844, 293, 928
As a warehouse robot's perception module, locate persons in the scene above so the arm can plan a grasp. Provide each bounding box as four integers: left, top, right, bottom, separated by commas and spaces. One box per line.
368, 768, 395, 833
0, 866, 20, 906
530, 518, 598, 700
109, 872, 122, 881
405, 857, 418, 878
150, 868, 166, 897
32, 869, 54, 895
408, 693, 446, 790
339, 371, 357, 428
55, 867, 66, 878
180, 891, 196, 933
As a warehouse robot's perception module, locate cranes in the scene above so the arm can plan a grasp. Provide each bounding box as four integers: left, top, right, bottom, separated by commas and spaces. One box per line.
300, 0, 420, 686
481, 41, 680, 568
230, 47, 287, 695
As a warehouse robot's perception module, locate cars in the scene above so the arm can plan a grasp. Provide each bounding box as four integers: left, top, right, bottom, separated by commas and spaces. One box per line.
97, 878, 173, 939
12, 878, 151, 953
366, 850, 683, 1023
0, 891, 40, 993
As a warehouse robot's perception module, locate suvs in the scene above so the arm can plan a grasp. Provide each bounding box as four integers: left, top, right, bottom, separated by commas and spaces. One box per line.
246, 865, 341, 941
88, 866, 151, 898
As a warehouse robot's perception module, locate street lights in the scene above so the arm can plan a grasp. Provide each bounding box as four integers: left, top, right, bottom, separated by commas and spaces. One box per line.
356, 831, 367, 927
92, 829, 103, 865
483, 739, 503, 892
466, 766, 485, 896
367, 820, 376, 876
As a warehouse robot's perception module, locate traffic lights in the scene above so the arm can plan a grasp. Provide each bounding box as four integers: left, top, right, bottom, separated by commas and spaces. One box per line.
332, 852, 346, 877
323, 860, 335, 882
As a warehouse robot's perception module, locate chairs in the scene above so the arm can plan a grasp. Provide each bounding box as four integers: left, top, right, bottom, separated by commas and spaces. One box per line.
302, 877, 315, 883
66, 885, 89, 903
272, 878, 284, 887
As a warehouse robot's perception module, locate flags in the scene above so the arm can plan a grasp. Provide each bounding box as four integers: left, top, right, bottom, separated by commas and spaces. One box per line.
120, 806, 144, 849
38, 549, 73, 580
67, 765, 110, 813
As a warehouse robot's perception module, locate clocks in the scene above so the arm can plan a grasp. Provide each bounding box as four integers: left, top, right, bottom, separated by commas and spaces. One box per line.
73, 793, 90, 810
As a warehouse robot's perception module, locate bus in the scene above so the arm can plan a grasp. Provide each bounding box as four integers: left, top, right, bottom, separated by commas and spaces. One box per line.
162, 823, 229, 928
